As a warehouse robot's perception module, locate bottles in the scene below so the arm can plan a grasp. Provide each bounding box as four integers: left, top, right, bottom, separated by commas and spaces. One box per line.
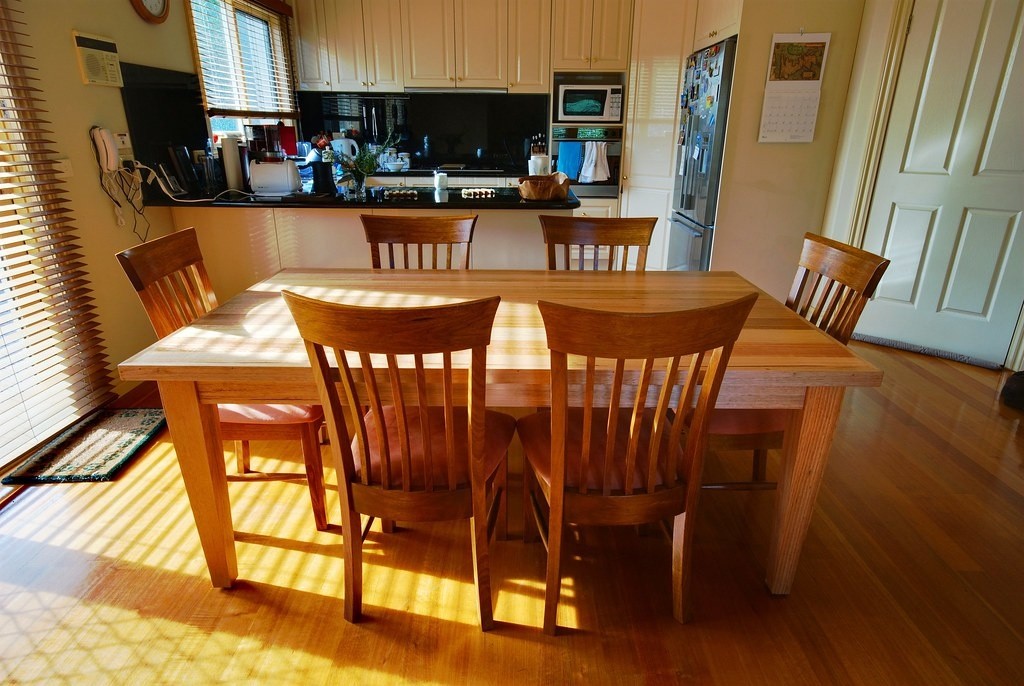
383, 147, 397, 167
397, 152, 411, 169
368, 146, 383, 167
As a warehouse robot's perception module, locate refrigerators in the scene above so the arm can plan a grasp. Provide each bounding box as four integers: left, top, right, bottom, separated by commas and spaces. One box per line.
667, 37, 736, 272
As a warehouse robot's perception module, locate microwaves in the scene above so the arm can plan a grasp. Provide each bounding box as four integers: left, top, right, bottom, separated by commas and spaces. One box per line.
558, 84, 622, 121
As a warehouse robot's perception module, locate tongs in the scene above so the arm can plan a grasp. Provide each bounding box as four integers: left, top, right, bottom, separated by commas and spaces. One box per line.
371, 107, 377, 142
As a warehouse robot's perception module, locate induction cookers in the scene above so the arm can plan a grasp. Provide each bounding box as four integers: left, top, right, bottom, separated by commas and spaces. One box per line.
433, 161, 505, 172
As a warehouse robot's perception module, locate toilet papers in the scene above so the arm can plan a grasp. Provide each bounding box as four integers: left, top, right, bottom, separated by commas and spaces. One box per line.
221, 137, 243, 194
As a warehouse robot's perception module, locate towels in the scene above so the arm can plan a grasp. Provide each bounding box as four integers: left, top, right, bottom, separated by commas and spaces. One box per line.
558, 141, 583, 180
580, 141, 610, 184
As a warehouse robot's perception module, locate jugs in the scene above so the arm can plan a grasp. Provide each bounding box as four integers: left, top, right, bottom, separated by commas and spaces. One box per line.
329, 139, 359, 172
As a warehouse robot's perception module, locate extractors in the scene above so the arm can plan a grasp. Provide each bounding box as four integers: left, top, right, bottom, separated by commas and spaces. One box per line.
405, 88, 508, 94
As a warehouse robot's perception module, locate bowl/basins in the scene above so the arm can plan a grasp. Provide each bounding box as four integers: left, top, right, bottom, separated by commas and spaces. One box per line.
386, 163, 404, 171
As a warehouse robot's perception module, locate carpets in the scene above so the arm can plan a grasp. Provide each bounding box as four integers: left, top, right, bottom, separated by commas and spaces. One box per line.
1, 400, 165, 482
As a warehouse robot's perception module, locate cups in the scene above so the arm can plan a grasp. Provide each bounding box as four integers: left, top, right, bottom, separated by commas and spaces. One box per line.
322, 146, 332, 162
305, 148, 321, 164
435, 189, 448, 202
316, 137, 327, 148
296, 142, 312, 155
528, 155, 550, 175
435, 174, 448, 189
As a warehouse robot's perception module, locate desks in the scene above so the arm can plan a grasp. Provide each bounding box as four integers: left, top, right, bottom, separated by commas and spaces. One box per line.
114, 262, 884, 600
142, 187, 582, 273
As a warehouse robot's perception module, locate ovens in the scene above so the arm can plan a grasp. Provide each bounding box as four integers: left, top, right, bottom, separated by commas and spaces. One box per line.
550, 125, 623, 196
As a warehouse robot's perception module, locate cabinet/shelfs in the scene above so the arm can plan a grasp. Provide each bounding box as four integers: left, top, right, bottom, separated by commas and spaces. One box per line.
572, 197, 618, 259
290, 0, 631, 95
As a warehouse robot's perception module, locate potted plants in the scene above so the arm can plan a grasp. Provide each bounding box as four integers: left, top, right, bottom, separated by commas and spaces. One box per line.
321, 121, 401, 203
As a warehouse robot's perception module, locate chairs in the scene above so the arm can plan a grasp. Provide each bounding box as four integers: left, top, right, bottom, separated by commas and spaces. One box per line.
514, 292, 759, 635
359, 214, 477, 413
278, 289, 516, 631
538, 216, 658, 273
662, 234, 891, 494
117, 228, 331, 530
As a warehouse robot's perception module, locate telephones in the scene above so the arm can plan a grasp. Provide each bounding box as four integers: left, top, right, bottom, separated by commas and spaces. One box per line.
157, 162, 188, 197
90, 127, 119, 173
